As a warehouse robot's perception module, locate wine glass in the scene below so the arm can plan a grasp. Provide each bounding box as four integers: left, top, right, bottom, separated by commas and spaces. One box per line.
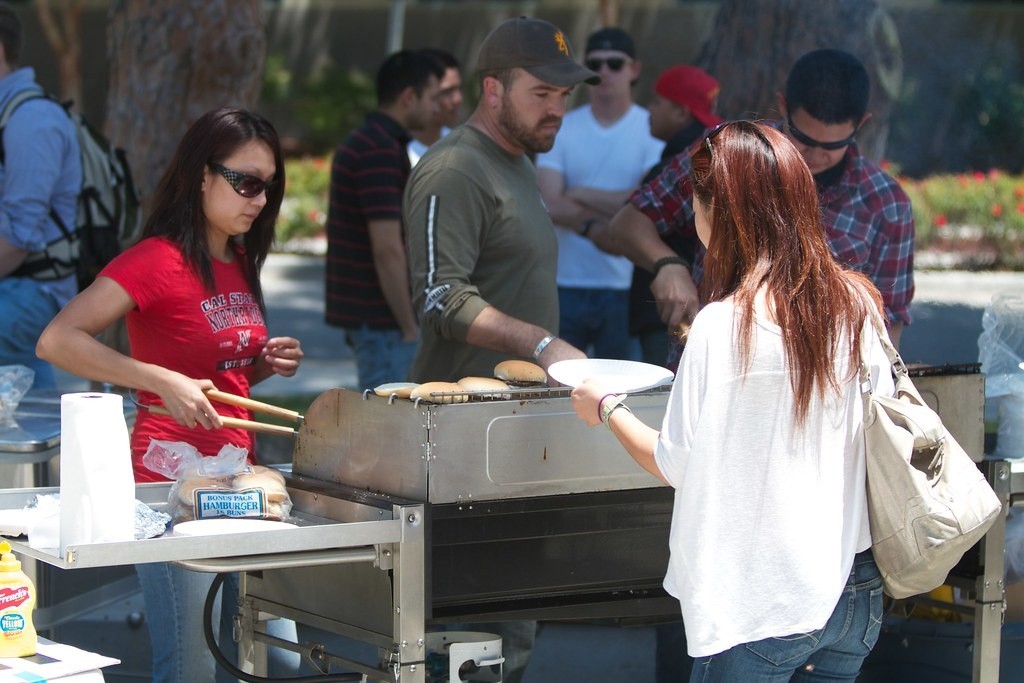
0, 364, 35, 432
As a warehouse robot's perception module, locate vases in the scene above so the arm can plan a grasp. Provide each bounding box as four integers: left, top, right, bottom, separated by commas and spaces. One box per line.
914, 230, 1024, 269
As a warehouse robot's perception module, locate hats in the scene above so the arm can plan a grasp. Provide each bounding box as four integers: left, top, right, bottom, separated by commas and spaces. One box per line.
584, 28, 641, 87
478, 16, 603, 86
656, 66, 723, 128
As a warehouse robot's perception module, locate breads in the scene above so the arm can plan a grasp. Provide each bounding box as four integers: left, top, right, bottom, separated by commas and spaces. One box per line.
176, 464, 291, 522
374, 376, 512, 403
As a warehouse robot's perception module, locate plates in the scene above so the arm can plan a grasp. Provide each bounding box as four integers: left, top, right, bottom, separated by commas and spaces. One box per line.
546, 357, 675, 396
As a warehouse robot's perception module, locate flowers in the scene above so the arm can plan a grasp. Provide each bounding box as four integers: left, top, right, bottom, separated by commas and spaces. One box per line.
876, 152, 1023, 238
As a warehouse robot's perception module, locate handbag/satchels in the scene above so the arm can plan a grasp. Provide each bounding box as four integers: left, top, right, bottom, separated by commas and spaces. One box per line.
845, 281, 1002, 600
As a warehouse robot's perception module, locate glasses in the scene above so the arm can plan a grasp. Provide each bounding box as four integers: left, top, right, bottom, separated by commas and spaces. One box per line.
206, 163, 282, 201
700, 118, 784, 157
585, 57, 625, 72
785, 97, 859, 151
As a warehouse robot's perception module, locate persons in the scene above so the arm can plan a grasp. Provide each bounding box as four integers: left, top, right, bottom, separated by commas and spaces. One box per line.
609, 51, 915, 358
404, 24, 724, 369
322, 49, 446, 390
36, 107, 305, 683
0, 5, 110, 644
406, 14, 586, 683
573, 108, 885, 683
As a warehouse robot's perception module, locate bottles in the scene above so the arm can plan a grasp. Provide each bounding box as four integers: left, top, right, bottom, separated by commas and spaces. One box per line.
0, 540, 39, 659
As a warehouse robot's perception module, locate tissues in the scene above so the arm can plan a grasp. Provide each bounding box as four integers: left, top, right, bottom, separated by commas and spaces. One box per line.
57, 390, 133, 559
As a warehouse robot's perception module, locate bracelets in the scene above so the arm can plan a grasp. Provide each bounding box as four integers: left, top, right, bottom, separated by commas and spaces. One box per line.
533, 334, 557, 360
603, 393, 633, 431
577, 187, 594, 211
651, 255, 689, 277
598, 392, 619, 423
580, 218, 596, 237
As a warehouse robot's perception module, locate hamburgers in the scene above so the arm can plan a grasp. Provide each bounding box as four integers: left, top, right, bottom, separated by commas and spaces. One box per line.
494, 360, 547, 397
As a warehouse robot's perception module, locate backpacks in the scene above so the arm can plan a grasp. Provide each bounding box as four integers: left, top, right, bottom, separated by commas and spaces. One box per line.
0, 88, 144, 286
